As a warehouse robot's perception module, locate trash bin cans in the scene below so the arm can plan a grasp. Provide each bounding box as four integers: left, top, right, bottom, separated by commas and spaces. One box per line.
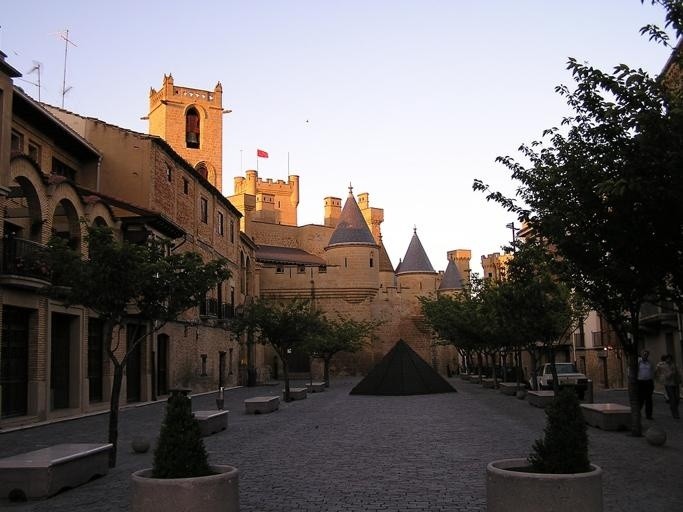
503, 365, 514, 382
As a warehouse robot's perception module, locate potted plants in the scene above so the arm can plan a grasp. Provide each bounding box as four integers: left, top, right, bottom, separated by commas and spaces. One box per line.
129, 390, 238, 510
485, 384, 602, 512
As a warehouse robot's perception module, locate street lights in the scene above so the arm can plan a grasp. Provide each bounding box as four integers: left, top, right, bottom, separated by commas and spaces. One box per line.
598, 343, 613, 389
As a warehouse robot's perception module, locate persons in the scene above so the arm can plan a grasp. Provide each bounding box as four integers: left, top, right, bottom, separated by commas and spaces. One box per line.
659, 353, 681, 419
654, 355, 669, 404
631, 349, 655, 420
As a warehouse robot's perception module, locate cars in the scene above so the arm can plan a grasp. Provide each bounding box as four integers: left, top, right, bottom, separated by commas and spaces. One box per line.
529, 362, 589, 400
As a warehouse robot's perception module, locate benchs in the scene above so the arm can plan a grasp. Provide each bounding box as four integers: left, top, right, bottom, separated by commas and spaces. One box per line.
1, 443, 114, 500
305, 383, 325, 392
457, 367, 634, 432
244, 396, 279, 414
192, 411, 229, 438
282, 387, 307, 401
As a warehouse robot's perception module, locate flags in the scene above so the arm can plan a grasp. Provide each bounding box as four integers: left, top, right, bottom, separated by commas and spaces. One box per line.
256, 149, 268, 159
